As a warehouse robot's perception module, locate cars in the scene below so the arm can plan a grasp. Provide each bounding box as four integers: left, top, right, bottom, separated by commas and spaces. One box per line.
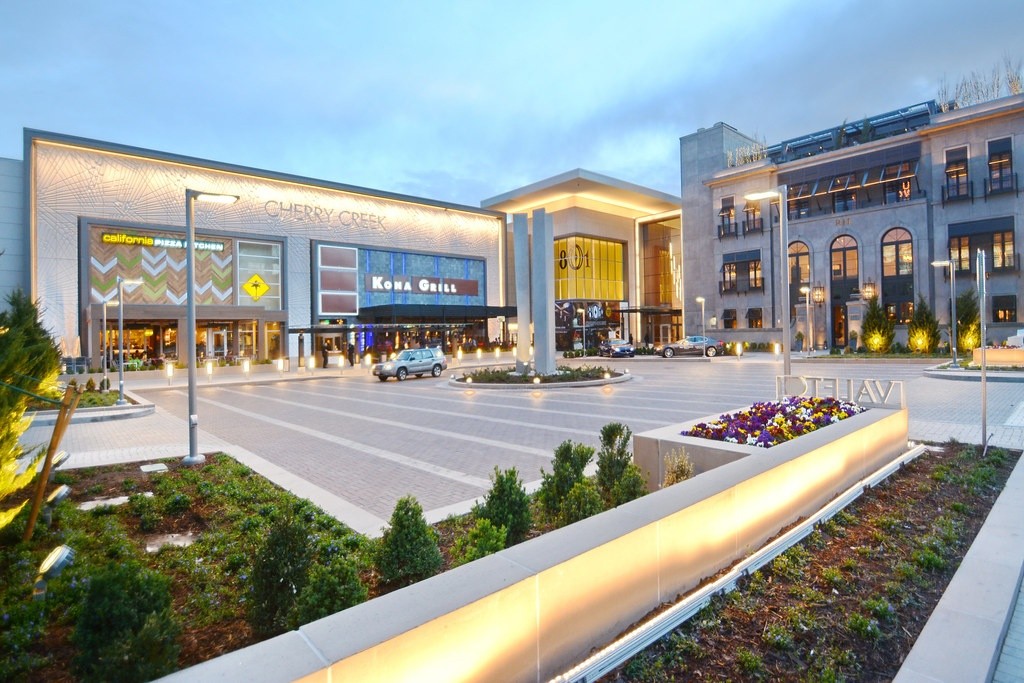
597, 338, 635, 358
653, 336, 726, 358
372, 348, 447, 381
431, 339, 446, 351
448, 341, 473, 352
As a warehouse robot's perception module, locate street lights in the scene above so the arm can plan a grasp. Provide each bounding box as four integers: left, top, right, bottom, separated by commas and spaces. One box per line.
577, 309, 586, 357
181, 188, 240, 464
101, 300, 119, 394
116, 280, 144, 405
696, 297, 707, 358
799, 287, 810, 356
745, 185, 791, 395
931, 260, 960, 368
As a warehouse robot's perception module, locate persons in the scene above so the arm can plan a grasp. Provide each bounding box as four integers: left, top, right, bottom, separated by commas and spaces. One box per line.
644, 334, 649, 346
629, 334, 633, 345
347, 344, 354, 366
322, 343, 329, 368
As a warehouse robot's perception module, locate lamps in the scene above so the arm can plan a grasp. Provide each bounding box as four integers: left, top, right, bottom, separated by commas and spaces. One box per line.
48, 449, 70, 481
32, 544, 75, 600
812, 281, 824, 307
41, 484, 72, 528
862, 277, 877, 301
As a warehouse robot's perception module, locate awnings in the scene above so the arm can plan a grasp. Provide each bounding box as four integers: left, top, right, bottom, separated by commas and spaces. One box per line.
993, 296, 1017, 311
745, 308, 762, 318
721, 310, 736, 320
